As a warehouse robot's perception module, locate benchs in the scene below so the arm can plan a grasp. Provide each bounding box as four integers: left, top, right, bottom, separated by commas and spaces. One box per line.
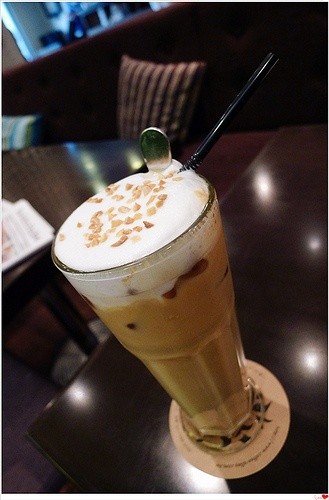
2, 349, 71, 494
2, 3, 328, 150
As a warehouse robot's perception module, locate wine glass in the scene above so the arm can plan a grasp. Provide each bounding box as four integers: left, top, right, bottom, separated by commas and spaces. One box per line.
51, 174, 264, 453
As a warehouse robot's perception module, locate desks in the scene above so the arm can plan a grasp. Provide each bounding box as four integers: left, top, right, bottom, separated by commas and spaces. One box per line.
2, 141, 140, 360
24, 126, 328, 494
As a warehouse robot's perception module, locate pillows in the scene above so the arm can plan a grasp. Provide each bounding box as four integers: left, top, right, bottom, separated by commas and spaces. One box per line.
116, 54, 207, 144
2, 114, 48, 151
